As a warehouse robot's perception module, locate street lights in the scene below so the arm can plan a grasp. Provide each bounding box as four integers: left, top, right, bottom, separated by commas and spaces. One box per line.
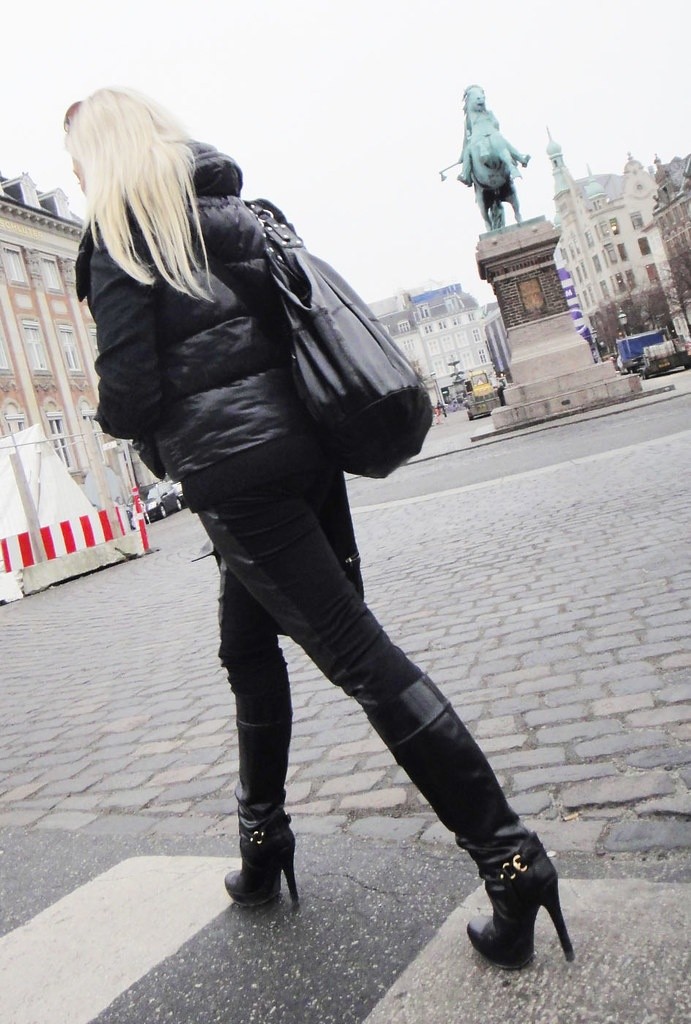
429, 372, 441, 404
591, 328, 605, 361
617, 306, 630, 336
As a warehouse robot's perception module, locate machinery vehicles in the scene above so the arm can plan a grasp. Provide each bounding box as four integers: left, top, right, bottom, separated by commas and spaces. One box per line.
464, 369, 505, 422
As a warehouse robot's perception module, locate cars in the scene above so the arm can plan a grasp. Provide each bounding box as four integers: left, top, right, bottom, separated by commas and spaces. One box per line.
168, 479, 187, 510
125, 481, 183, 528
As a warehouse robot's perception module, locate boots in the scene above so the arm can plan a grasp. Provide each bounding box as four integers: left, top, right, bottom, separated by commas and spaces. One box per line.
367, 672, 579, 969
224, 717, 301, 908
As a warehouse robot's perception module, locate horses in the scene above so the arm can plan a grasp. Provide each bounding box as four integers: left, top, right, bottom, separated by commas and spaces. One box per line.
456, 86, 531, 226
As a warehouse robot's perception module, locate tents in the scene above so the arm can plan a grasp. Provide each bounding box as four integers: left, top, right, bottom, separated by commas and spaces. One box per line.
1, 423, 139, 603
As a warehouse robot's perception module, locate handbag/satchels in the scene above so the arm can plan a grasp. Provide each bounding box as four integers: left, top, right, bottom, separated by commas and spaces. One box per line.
241, 197, 434, 479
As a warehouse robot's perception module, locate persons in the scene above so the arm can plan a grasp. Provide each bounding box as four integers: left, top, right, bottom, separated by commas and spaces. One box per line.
66, 87, 574, 972
457, 106, 532, 186
